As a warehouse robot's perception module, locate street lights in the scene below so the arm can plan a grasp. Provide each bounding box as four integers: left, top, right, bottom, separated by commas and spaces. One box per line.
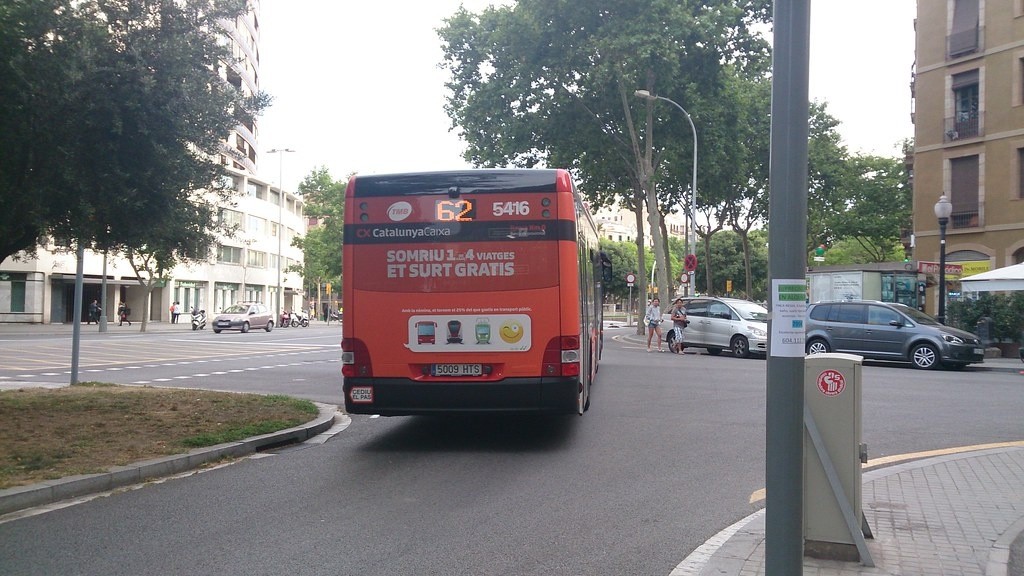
266, 149, 295, 326
634, 91, 698, 294
934, 192, 952, 324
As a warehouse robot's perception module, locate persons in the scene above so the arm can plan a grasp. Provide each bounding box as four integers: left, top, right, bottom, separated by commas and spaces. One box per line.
170, 302, 181, 324
118, 298, 131, 326
646, 298, 665, 352
88, 298, 102, 325
671, 299, 686, 355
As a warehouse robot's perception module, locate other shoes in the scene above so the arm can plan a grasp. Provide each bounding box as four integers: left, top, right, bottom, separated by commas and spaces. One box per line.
647, 347, 651, 353
673, 346, 678, 353
129, 323, 131, 326
679, 351, 685, 355
658, 348, 665, 352
118, 324, 122, 326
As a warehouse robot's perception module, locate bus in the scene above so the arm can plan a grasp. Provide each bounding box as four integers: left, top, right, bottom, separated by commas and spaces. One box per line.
341, 168, 613, 421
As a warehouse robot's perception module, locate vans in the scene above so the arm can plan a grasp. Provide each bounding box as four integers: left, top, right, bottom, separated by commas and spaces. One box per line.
659, 293, 768, 358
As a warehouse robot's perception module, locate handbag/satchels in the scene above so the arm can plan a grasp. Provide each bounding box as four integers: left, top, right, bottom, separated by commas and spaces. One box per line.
643, 316, 651, 327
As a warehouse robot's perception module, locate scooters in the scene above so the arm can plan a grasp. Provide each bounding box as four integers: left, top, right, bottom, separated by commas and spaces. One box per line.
190, 306, 207, 330
278, 306, 313, 328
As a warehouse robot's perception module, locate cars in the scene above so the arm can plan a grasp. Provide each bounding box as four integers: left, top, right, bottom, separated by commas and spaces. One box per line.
331, 315, 338, 321
211, 302, 274, 333
806, 299, 984, 370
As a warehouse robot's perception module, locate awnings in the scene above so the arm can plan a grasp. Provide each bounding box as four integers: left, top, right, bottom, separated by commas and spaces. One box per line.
958, 262, 1024, 292
